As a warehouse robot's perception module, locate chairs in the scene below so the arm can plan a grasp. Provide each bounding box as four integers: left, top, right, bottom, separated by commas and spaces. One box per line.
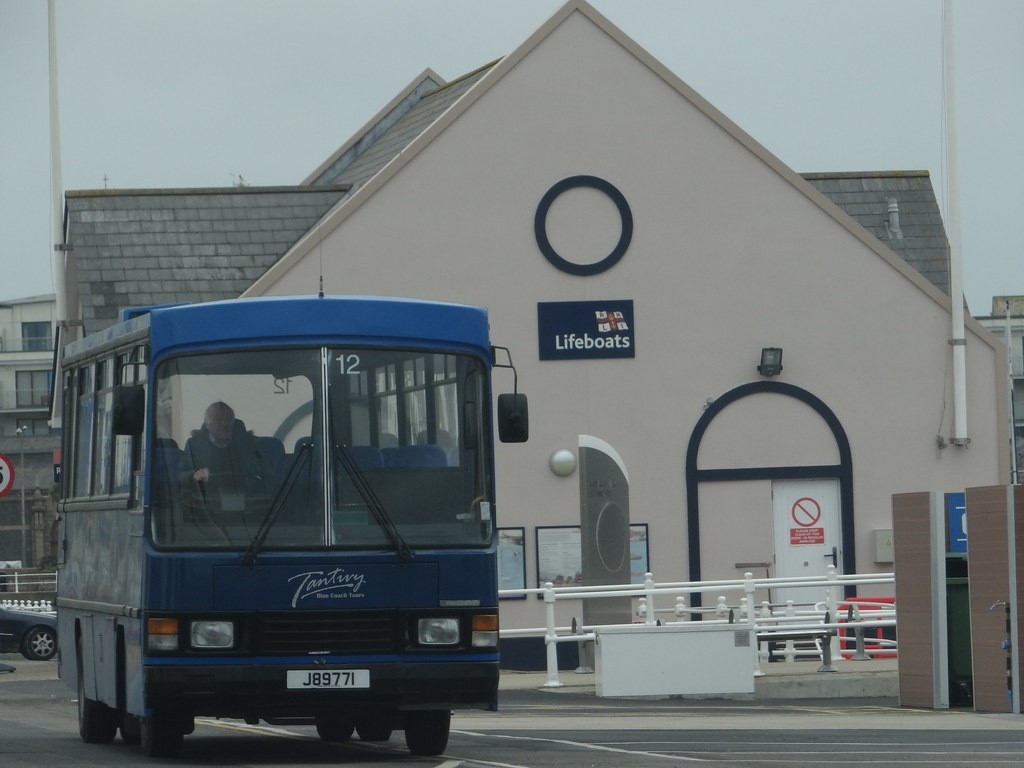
154, 437, 452, 510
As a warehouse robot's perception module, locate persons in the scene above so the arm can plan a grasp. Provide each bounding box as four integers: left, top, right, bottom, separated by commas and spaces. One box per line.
176, 401, 264, 486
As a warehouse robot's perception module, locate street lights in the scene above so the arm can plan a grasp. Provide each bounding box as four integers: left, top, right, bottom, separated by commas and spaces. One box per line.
16, 424, 29, 565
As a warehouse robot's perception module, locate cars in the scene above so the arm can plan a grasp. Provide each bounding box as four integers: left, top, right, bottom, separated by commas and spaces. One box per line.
0, 601, 58, 662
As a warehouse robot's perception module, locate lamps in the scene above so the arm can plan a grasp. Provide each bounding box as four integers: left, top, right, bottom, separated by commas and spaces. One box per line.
756, 346, 783, 378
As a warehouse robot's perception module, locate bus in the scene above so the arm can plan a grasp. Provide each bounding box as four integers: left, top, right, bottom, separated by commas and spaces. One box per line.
45, 294, 530, 756
